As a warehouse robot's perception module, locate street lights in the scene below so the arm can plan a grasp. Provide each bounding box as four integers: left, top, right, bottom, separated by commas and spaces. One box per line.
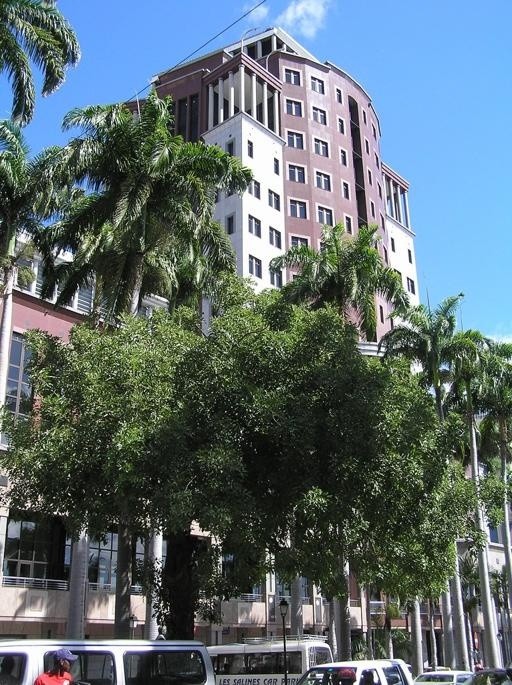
128, 614, 139, 639
278, 598, 289, 685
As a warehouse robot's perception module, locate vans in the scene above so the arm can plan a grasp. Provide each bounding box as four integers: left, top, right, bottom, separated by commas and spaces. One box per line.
0, 639, 215, 685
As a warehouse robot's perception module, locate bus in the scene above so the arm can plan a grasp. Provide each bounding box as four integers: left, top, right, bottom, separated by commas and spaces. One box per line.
191, 639, 335, 685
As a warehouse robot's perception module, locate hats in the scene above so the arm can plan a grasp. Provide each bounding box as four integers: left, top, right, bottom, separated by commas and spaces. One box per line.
53, 649, 79, 661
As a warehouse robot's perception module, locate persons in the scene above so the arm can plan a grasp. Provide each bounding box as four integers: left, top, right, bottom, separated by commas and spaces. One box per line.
33, 648, 79, 685
475, 659, 484, 672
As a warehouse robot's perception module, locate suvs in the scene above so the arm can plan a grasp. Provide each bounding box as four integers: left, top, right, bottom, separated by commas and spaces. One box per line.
296, 659, 415, 685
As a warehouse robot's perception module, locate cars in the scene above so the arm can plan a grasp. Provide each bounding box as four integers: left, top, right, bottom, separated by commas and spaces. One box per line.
414, 667, 512, 685
